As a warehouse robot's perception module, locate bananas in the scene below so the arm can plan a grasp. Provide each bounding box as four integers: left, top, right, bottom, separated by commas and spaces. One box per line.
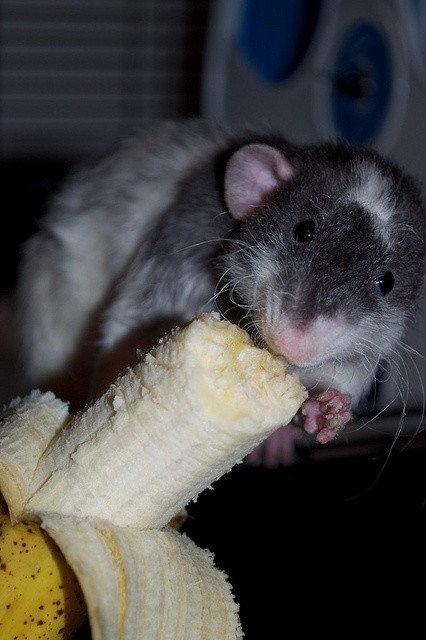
0, 313, 309, 640
